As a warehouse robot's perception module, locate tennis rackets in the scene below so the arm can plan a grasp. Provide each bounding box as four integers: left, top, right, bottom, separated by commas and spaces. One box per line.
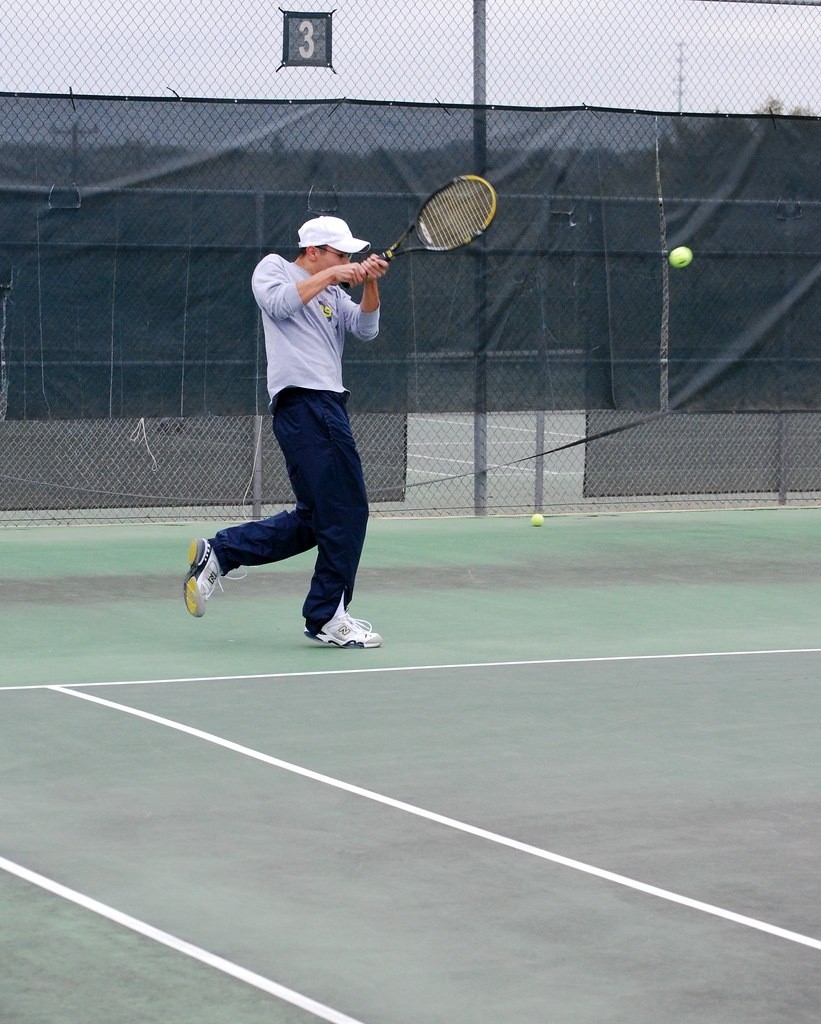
340, 175, 498, 290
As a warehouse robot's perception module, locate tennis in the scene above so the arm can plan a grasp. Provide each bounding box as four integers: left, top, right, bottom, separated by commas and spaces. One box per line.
531, 514, 544, 525
668, 246, 693, 268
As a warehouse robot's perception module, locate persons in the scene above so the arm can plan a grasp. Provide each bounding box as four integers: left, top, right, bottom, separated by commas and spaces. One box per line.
183, 215, 390, 648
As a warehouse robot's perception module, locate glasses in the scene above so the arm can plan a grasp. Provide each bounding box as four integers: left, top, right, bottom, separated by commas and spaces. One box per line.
315, 246, 352, 263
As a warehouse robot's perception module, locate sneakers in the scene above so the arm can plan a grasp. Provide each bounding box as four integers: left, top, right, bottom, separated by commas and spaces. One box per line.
303, 604, 383, 649
183, 536, 247, 617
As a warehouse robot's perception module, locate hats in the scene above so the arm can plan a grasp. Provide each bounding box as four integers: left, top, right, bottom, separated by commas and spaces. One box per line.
297, 216, 371, 254
